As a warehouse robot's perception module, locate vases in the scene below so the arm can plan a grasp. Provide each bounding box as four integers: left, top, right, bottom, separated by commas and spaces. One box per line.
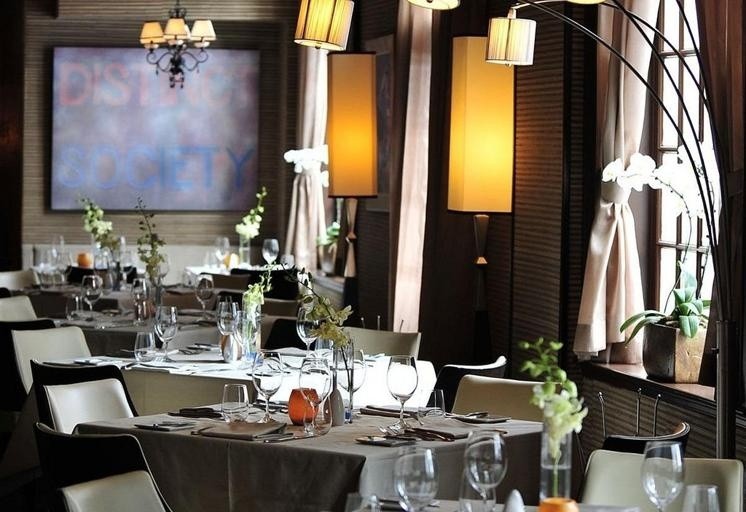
237, 235, 250, 266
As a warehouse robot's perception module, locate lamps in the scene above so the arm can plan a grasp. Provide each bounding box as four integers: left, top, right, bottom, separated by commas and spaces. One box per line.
448, 34, 513, 214
293, 1, 736, 460
325, 52, 379, 198
137, 0, 218, 88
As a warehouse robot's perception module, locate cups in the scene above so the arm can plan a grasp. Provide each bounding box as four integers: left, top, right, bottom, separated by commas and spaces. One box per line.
281, 254, 293, 270
421, 388, 445, 422
344, 493, 382, 512
393, 446, 440, 512
459, 467, 497, 512
683, 483, 720, 511
217, 302, 259, 368
223, 384, 250, 424
134, 331, 155, 363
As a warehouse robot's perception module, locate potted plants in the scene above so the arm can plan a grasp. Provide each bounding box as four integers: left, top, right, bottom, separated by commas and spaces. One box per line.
619, 260, 712, 383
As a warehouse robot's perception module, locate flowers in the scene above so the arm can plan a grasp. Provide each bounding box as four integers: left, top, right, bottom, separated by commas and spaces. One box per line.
599, 140, 723, 316
236, 187, 268, 242
79, 197, 115, 253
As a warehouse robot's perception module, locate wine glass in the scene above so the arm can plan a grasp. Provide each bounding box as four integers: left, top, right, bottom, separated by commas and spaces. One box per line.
40, 231, 148, 328
467, 428, 508, 512
215, 234, 277, 271
254, 307, 419, 440
641, 441, 684, 512
195, 276, 217, 324
155, 307, 178, 364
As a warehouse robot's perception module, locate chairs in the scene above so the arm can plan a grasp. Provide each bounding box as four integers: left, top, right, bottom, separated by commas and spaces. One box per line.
327, 325, 422, 363
451, 374, 564, 422
9, 326, 92, 394
581, 449, 744, 512
41, 379, 133, 433
59, 470, 165, 512
265, 318, 329, 351
36, 422, 173, 510
426, 355, 506, 414
30, 358, 140, 422
